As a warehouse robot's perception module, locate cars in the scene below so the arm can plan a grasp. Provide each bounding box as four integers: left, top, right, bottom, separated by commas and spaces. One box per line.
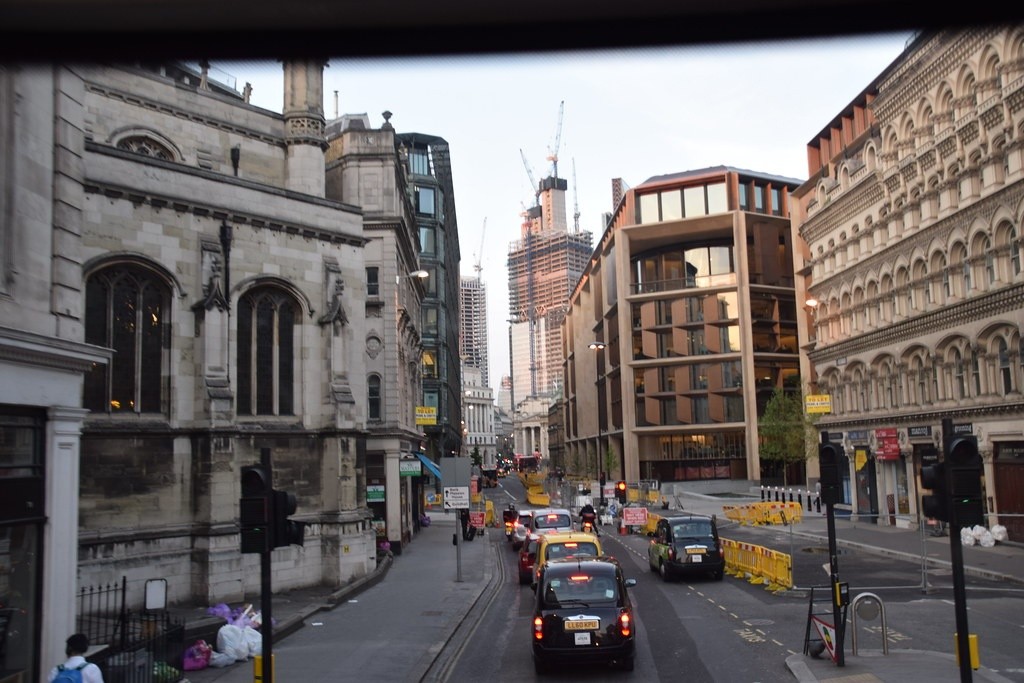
518, 531, 553, 584
498, 471, 506, 477
513, 510, 532, 550
532, 555, 637, 672
531, 532, 603, 588
647, 515, 725, 582
524, 510, 575, 535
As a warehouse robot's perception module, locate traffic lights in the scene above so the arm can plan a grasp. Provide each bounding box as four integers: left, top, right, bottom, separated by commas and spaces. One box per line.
817, 442, 844, 504
943, 430, 983, 529
272, 488, 306, 548
920, 462, 948, 522
237, 464, 273, 554
615, 480, 626, 504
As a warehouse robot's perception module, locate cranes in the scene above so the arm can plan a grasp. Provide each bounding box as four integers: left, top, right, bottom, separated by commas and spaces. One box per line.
519, 148, 544, 205
570, 156, 582, 233
472, 217, 488, 281
547, 99, 564, 178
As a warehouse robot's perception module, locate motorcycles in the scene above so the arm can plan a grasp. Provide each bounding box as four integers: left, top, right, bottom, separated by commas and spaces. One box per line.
505, 518, 514, 541
580, 512, 595, 533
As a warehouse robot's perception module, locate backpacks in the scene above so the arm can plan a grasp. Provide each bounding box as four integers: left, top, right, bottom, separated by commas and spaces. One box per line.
51, 662, 89, 683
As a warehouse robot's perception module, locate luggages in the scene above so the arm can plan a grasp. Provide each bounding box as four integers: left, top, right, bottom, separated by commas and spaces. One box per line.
467, 525, 476, 541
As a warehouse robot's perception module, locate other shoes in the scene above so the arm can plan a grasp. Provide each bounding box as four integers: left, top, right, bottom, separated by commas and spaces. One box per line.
597, 534, 600, 537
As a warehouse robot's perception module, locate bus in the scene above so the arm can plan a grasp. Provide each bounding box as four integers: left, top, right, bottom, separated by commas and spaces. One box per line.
481, 468, 498, 488
519, 456, 538, 473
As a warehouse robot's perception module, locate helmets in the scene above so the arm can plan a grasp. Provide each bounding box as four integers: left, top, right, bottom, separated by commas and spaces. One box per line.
585, 499, 590, 505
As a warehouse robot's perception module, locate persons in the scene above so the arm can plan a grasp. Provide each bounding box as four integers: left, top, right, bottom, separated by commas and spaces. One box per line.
813, 479, 821, 506
579, 499, 601, 537
461, 508, 471, 540
48, 633, 104, 683
506, 503, 519, 522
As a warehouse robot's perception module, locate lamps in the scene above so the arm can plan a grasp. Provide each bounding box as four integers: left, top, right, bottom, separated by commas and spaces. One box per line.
398, 270, 429, 279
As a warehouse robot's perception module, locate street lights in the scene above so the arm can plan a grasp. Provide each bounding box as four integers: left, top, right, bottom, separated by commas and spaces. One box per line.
588, 341, 607, 505
